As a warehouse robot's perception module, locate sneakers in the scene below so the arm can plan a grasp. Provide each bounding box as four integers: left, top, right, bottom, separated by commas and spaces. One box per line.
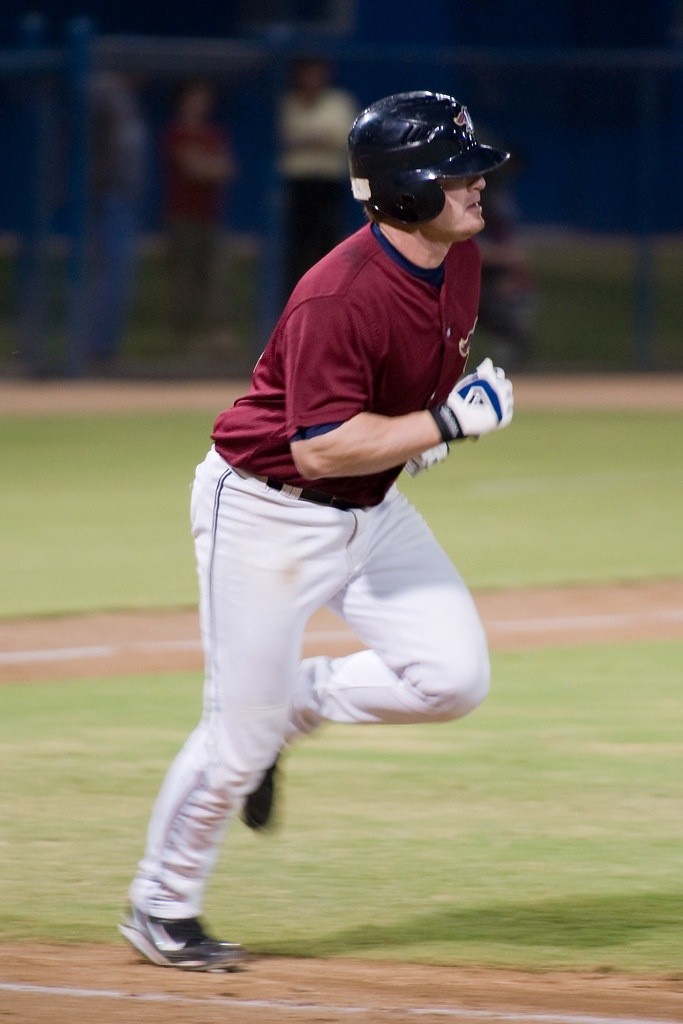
234, 764, 277, 830
115, 905, 248, 972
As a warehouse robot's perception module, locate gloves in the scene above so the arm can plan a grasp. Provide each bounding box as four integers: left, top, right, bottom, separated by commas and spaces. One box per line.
430, 356, 515, 442
404, 441, 450, 476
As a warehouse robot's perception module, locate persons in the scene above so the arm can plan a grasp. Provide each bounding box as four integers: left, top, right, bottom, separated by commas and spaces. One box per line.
116, 88, 514, 972
162, 51, 362, 364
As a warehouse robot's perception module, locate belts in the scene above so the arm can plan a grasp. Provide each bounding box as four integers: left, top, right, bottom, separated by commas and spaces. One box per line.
266, 480, 364, 511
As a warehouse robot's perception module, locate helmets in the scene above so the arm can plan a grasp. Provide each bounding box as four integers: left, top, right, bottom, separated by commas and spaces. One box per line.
346, 90, 512, 223
